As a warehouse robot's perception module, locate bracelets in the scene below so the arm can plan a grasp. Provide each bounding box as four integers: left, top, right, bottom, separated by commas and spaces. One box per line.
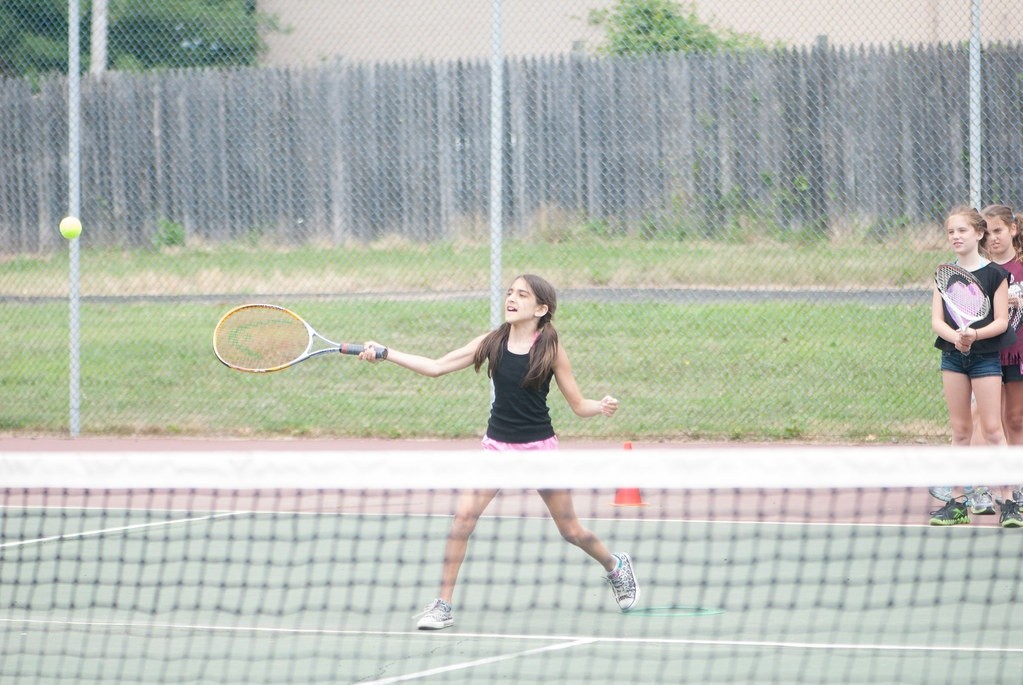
973, 328, 977, 342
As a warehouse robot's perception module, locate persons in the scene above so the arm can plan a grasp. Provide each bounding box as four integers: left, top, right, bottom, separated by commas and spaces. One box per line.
359, 276, 640, 630
929, 204, 1023, 527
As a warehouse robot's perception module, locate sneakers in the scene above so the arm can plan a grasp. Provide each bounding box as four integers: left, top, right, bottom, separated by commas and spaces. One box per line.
929, 495, 971, 526
1013, 489, 1023, 512
994, 496, 1002, 505
600, 552, 640, 613
412, 599, 455, 629
970, 487, 996, 514
929, 487, 975, 507
999, 498, 1023, 527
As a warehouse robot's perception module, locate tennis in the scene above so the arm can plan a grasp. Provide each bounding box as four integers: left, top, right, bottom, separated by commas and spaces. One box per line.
59, 216, 83, 240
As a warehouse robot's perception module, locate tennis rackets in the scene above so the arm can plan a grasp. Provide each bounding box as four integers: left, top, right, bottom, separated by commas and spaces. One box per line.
935, 263, 991, 357
212, 304, 388, 374
1007, 283, 1023, 333
